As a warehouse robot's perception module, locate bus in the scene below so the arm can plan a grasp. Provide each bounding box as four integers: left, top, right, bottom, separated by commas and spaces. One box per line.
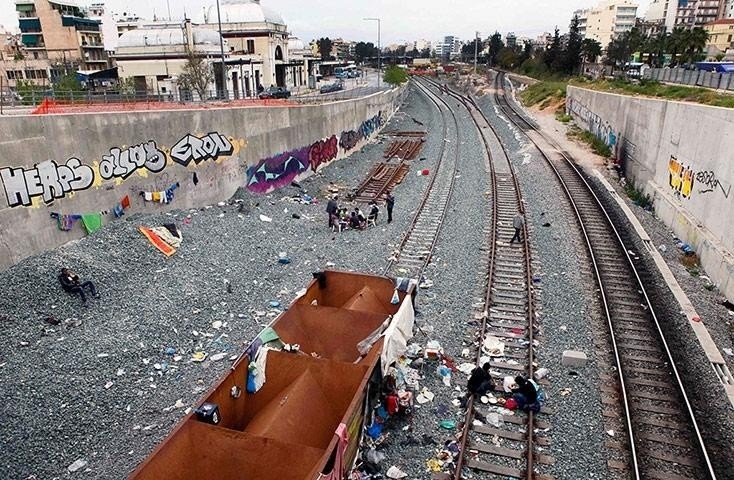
333, 66, 357, 79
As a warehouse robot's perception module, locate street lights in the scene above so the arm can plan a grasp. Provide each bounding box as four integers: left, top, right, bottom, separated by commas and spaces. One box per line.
360, 16, 382, 93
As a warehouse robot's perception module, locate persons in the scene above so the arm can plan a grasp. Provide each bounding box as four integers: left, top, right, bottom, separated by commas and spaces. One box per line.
586, 66, 607, 78
326, 194, 379, 231
466, 362, 493, 395
689, 61, 699, 71
711, 67, 717, 74
509, 211, 525, 244
58, 267, 101, 308
258, 84, 264, 92
507, 375, 537, 410
384, 387, 414, 418
383, 190, 394, 223
438, 83, 450, 95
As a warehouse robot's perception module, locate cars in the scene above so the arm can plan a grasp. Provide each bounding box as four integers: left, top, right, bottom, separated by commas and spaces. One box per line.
320, 85, 335, 93
258, 86, 292, 100
